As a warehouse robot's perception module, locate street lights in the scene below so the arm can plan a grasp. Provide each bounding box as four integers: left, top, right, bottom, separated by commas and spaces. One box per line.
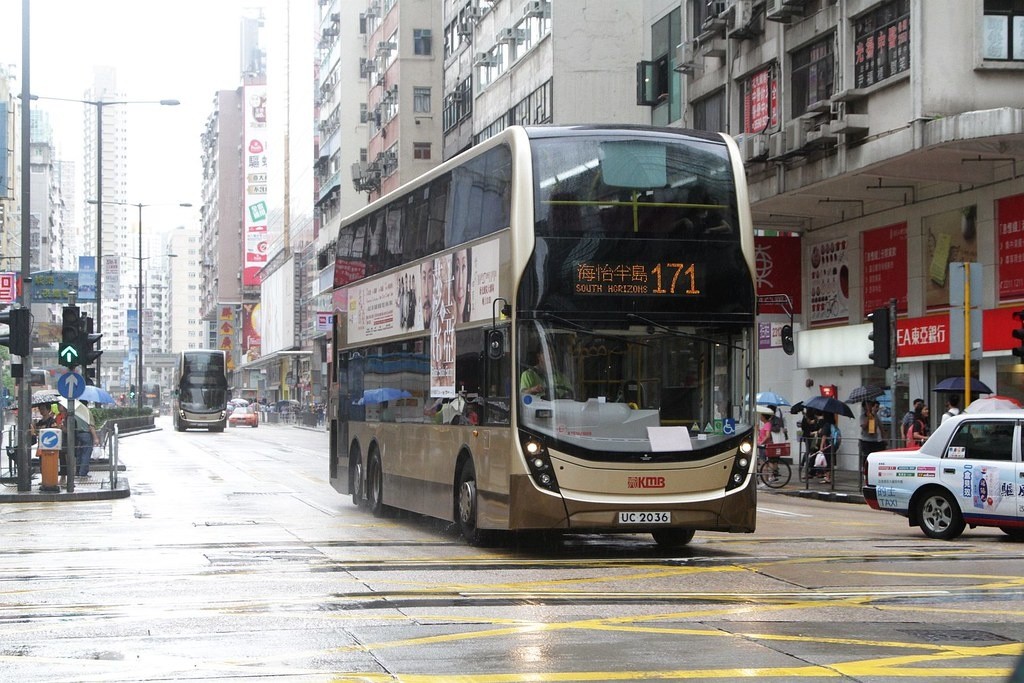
110, 285, 170, 400
87, 199, 194, 408
103, 252, 180, 403
18, 94, 182, 419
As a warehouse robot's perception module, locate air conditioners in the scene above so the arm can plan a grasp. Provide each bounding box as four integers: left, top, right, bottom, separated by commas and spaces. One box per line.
733, 118, 816, 164
726, 0, 752, 35
675, 40, 694, 66
467, 6, 481, 18
767, 0, 804, 18
473, 52, 489, 66
523, 0, 544, 19
364, 8, 374, 18
495, 27, 515, 45
456, 22, 472, 35
448, 91, 462, 101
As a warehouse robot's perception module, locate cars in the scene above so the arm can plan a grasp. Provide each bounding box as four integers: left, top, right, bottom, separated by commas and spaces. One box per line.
228, 407, 260, 427
226, 398, 300, 415
862, 408, 1023, 541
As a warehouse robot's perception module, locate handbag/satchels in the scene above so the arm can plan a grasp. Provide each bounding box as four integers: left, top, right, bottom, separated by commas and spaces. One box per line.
771, 427, 785, 443
869, 418, 875, 434
810, 439, 818, 450
814, 452, 827, 468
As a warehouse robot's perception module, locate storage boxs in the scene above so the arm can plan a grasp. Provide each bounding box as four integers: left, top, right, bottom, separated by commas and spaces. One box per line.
765, 443, 790, 456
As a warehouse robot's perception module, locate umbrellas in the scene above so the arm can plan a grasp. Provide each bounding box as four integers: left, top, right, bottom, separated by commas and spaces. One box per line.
351, 388, 413, 406
79, 386, 115, 404
3, 396, 62, 410
932, 376, 993, 394
845, 385, 886, 404
34, 389, 60, 396
57, 396, 95, 426
790, 401, 804, 414
745, 404, 774, 414
277, 399, 299, 405
746, 392, 791, 407
801, 395, 855, 419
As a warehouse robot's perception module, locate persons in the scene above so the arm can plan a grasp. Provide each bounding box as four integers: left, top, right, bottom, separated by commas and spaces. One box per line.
419, 260, 435, 329
520, 341, 571, 399
900, 398, 929, 446
755, 413, 772, 487
395, 272, 417, 330
379, 401, 396, 423
941, 395, 960, 426
13, 400, 99, 488
767, 405, 788, 484
667, 187, 732, 235
450, 247, 472, 324
280, 403, 328, 426
801, 409, 837, 484
858, 399, 888, 467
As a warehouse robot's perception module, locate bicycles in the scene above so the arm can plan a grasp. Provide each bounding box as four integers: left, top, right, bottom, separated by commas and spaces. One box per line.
757, 445, 792, 489
798, 434, 818, 484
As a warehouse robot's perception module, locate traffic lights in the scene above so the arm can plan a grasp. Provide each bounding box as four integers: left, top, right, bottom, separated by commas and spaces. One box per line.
80, 329, 104, 366
0, 306, 31, 357
129, 384, 135, 399
1011, 308, 1024, 368
865, 307, 891, 370
57, 305, 81, 368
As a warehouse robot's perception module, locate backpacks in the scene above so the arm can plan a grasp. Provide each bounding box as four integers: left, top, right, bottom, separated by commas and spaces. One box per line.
906, 420, 924, 448
830, 425, 841, 448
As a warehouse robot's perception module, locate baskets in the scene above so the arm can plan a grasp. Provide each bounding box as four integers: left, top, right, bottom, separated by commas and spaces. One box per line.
766, 442, 790, 457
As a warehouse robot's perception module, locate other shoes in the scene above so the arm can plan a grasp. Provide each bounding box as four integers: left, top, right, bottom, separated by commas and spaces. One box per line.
819, 478, 831, 483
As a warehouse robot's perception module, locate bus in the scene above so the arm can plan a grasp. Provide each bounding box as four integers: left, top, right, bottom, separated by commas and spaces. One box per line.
172, 348, 233, 433
14, 366, 51, 405
327, 127, 796, 548
